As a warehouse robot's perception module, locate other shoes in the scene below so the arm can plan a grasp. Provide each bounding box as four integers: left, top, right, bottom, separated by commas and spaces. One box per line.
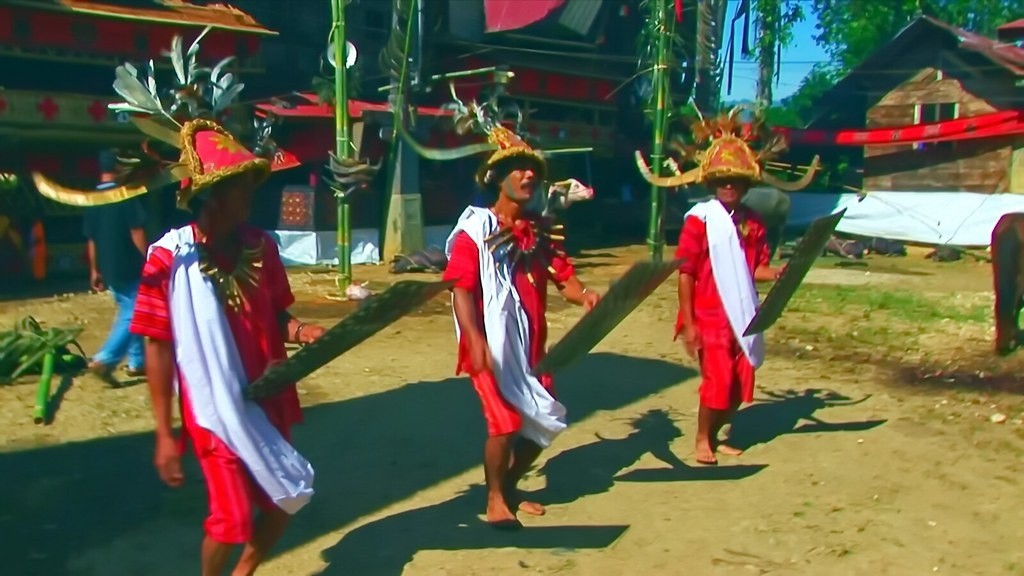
87, 360, 123, 388
122, 365, 144, 377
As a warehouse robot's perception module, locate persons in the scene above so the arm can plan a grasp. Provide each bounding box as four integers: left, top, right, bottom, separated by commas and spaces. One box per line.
672, 139, 789, 465
129, 132, 330, 576
82, 148, 152, 388
441, 126, 603, 531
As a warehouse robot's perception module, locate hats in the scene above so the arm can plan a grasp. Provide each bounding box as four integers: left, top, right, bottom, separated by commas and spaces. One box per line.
27, 22, 283, 209
636, 91, 820, 193
393, 76, 595, 190
